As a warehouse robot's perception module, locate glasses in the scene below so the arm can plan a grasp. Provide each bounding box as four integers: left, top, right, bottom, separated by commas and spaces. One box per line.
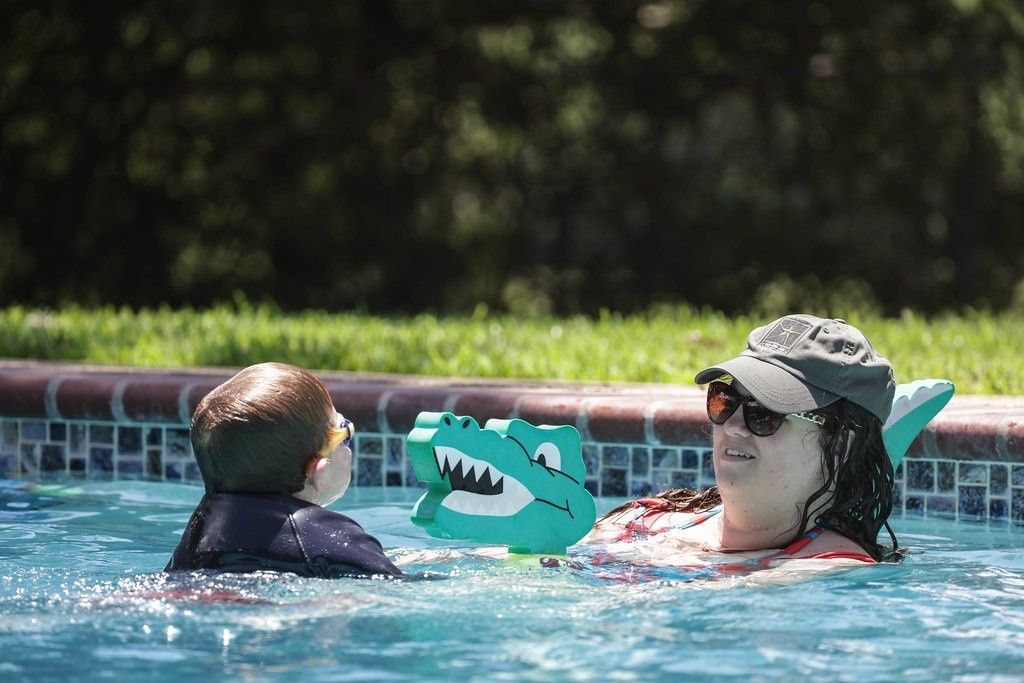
706, 381, 840, 437
304, 417, 355, 476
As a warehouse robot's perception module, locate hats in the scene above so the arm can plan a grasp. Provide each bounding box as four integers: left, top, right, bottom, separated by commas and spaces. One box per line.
694, 314, 896, 427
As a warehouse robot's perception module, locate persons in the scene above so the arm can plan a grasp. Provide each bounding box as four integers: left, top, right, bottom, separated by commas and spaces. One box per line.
158, 361, 405, 580
568, 314, 924, 565
